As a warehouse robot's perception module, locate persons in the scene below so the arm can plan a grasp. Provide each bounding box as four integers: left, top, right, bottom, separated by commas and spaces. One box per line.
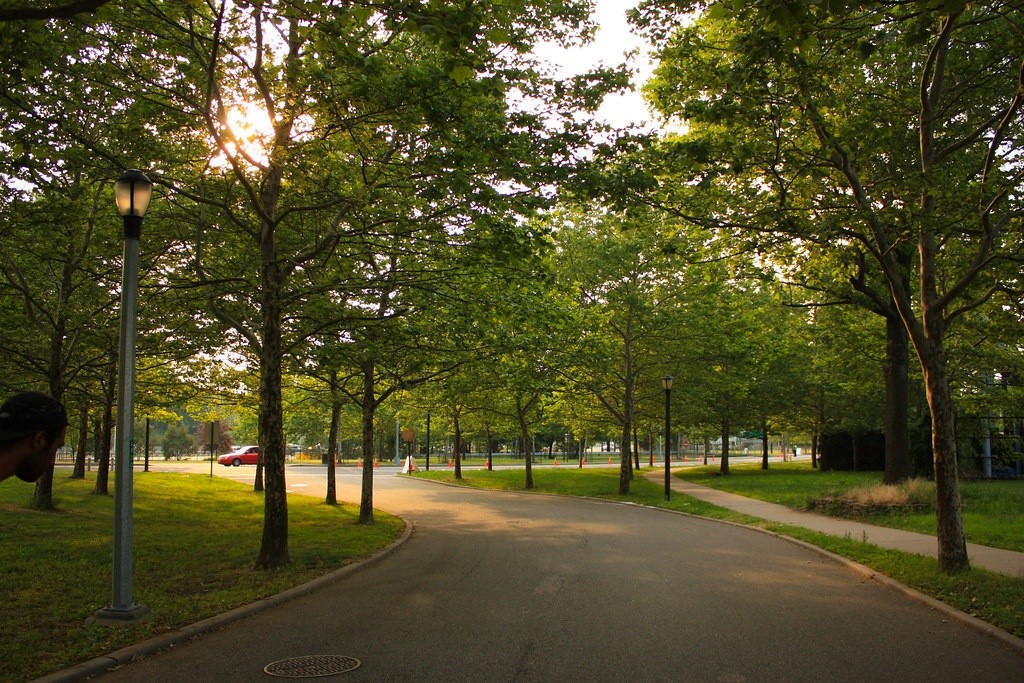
744, 446, 749, 456
792, 445, 796, 457
0, 392, 68, 483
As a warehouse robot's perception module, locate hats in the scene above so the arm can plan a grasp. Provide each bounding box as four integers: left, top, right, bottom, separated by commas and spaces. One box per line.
1, 392, 67, 439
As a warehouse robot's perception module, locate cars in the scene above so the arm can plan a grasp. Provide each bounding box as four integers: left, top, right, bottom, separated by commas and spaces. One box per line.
600, 442, 619, 451
217, 446, 264, 466
304, 443, 328, 455
286, 443, 303, 456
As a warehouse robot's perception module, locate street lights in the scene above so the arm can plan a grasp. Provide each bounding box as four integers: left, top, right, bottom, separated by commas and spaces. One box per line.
660, 375, 675, 501
94, 169, 152, 619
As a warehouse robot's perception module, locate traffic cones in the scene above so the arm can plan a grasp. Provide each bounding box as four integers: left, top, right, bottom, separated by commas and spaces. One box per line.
608, 455, 612, 463
449, 459, 453, 468
700, 455, 703, 463
486, 457, 489, 467
374, 457, 378, 468
357, 457, 362, 467
554, 457, 557, 465
685, 455, 688, 462
582, 457, 584, 464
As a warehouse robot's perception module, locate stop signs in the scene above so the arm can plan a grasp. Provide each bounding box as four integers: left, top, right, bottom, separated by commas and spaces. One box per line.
683, 441, 689, 448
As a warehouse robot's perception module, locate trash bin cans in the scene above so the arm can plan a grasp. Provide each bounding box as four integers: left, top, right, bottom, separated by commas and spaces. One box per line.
322, 454, 327, 463
